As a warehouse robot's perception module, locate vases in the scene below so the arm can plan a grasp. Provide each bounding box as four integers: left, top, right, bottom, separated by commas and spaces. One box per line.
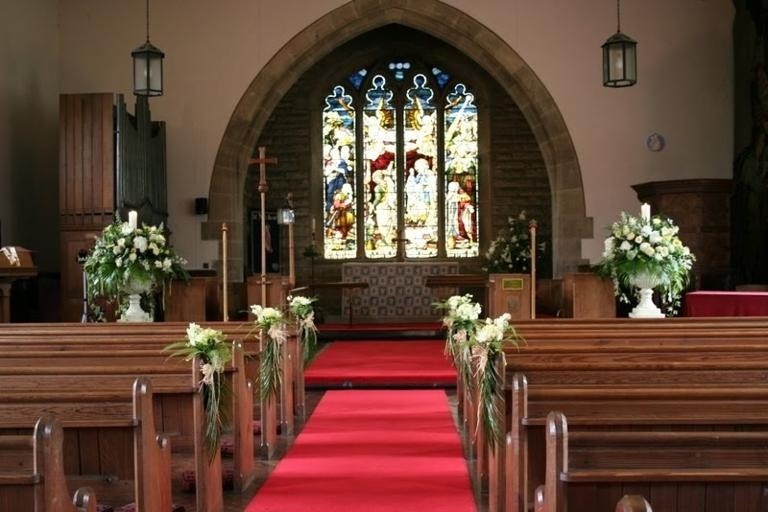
116, 294, 152, 323
628, 288, 665, 318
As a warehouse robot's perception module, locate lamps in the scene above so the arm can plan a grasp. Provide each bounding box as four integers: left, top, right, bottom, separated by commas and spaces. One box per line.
130, 0, 164, 97
601, 1, 640, 87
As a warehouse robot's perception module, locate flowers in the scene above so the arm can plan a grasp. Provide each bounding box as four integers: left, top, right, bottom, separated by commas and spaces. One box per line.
483, 210, 546, 273
430, 293, 482, 354
77, 208, 194, 316
599, 212, 697, 318
288, 294, 318, 341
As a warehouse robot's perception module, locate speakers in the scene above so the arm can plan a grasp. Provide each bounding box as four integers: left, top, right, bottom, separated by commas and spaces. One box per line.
195, 198, 207, 215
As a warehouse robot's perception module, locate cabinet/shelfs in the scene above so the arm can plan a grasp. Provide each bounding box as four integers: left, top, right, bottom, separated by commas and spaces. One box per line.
247, 273, 289, 321
58, 91, 167, 322
630, 178, 734, 290
564, 272, 617, 318
164, 275, 219, 322
486, 273, 530, 320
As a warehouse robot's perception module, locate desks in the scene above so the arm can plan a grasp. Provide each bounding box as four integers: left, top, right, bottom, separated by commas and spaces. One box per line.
686, 291, 768, 317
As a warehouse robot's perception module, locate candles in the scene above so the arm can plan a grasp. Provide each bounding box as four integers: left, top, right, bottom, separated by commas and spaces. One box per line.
129, 209, 138, 228
641, 202, 652, 223
